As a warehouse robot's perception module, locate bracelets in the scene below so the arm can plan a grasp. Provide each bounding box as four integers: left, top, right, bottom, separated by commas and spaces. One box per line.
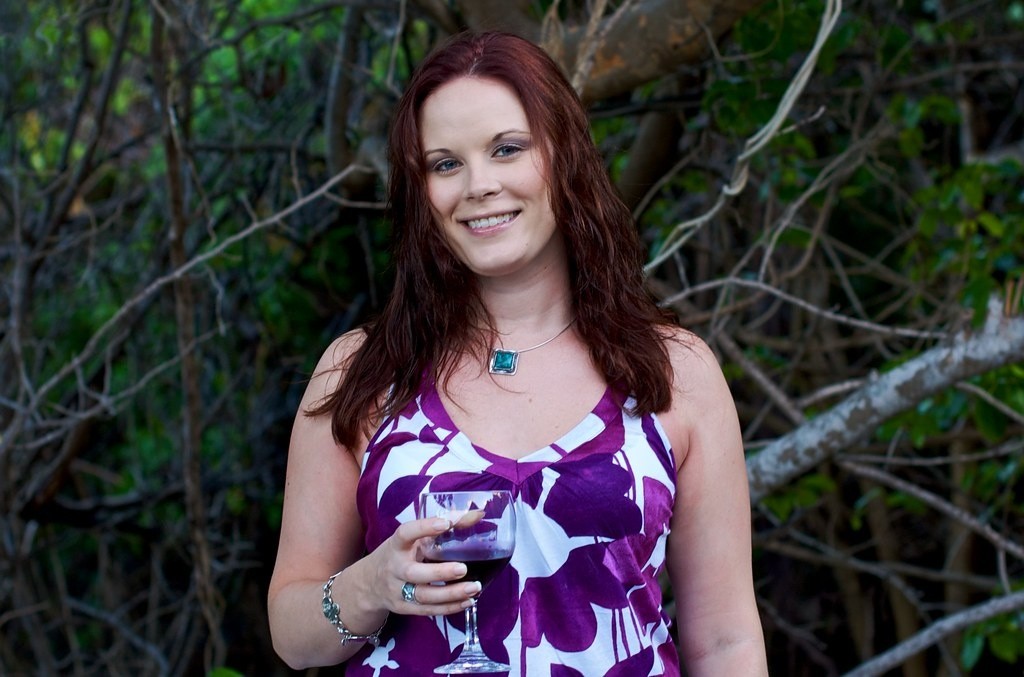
322, 570, 389, 647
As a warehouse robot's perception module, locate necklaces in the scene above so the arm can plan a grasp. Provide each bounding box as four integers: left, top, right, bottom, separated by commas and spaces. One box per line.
467, 313, 580, 376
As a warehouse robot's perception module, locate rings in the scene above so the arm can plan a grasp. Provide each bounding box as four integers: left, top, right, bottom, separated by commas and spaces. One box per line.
401, 582, 421, 605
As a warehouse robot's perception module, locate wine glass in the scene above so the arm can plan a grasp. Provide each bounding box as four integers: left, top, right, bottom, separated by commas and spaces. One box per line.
417, 491, 517, 674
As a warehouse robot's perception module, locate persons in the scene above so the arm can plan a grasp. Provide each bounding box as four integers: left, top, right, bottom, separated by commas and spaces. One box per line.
268, 28, 770, 677
